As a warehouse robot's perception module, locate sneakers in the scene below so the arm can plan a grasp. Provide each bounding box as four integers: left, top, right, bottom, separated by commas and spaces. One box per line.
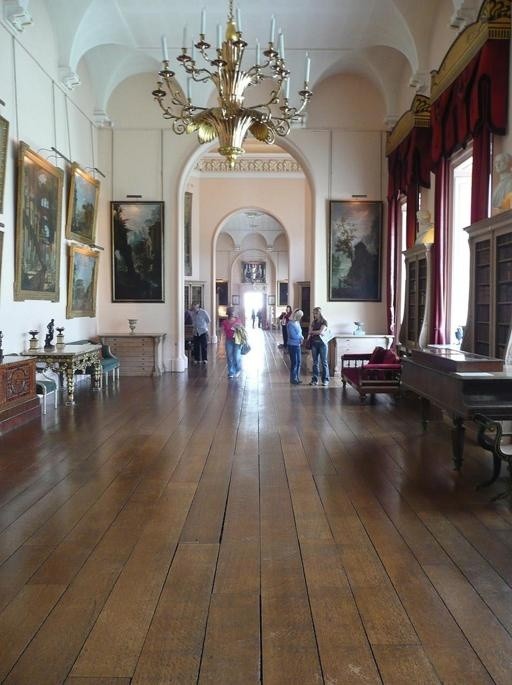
323, 381, 328, 386
309, 381, 318, 385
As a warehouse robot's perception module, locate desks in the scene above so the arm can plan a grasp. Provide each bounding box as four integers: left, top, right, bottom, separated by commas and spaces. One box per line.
19, 344, 104, 408
0, 356, 41, 436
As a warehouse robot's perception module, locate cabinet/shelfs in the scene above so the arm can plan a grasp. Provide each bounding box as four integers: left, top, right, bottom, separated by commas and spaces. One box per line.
328, 334, 396, 378
95, 336, 167, 377
183, 280, 206, 356
399, 244, 433, 352
461, 207, 511, 365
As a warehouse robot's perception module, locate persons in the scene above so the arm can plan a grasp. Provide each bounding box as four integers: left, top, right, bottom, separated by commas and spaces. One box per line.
286, 308, 305, 384
243, 264, 263, 283
188, 300, 211, 364
221, 306, 247, 378
251, 310, 256, 328
256, 309, 262, 328
304, 307, 331, 386
278, 304, 293, 354
414, 208, 434, 245
491, 152, 512, 210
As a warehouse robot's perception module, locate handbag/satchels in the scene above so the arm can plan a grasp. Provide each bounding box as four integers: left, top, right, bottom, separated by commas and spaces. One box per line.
241, 344, 250, 354
306, 339, 311, 349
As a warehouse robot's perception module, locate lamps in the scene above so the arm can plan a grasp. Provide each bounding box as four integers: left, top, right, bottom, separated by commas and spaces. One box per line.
151, 0, 313, 172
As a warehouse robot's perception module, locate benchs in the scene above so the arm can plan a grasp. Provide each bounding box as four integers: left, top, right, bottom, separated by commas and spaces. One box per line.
340, 346, 403, 401
65, 340, 121, 384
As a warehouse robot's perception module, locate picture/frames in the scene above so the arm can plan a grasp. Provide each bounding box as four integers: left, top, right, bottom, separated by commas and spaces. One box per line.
0, 113, 166, 320
327, 200, 384, 302
183, 190, 194, 276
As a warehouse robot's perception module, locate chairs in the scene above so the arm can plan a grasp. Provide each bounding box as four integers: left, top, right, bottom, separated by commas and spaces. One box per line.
1, 352, 60, 415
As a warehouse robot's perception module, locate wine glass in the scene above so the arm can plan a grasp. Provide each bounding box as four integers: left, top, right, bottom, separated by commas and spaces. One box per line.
454, 327, 463, 346
129, 319, 138, 335
353, 320, 365, 335
